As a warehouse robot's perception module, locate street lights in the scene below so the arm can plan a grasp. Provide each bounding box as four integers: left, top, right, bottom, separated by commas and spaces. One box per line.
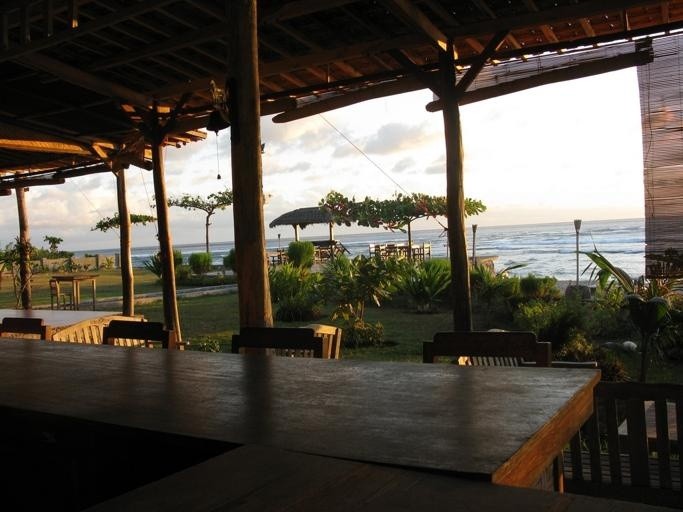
573, 218, 583, 287
470, 222, 480, 266
277, 233, 282, 248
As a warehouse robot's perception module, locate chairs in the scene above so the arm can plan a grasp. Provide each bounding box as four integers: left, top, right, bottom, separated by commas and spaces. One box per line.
422, 331, 553, 368
313, 241, 351, 264
367, 239, 433, 261
231, 327, 323, 358
49, 279, 73, 310
266, 248, 290, 266
0, 318, 51, 341
102, 320, 176, 349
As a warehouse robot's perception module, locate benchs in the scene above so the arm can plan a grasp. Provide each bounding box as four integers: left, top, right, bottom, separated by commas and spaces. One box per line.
53, 315, 190, 350
275, 325, 342, 359
458, 329, 598, 370
554, 381, 682, 509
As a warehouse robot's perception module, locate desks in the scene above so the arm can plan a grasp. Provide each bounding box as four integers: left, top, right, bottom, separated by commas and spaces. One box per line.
52, 274, 101, 311
